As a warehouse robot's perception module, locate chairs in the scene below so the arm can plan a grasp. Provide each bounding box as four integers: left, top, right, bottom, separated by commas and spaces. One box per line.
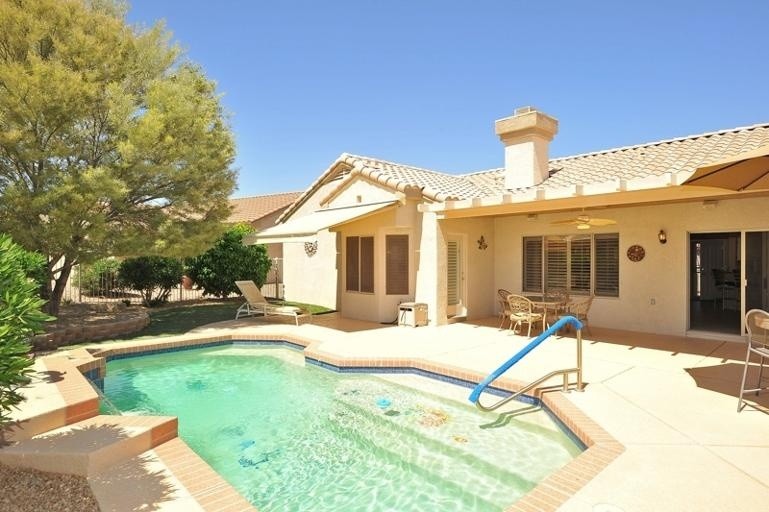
731, 306, 769, 415
230, 278, 315, 328
490, 283, 596, 344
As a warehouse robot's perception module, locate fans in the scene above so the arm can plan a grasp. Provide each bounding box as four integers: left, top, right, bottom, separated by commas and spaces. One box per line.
545, 207, 617, 231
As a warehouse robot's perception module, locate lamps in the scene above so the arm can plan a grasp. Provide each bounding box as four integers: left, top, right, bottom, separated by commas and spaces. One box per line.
658, 229, 668, 244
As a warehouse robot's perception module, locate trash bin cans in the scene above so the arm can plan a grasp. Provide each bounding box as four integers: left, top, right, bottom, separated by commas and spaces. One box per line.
397, 302, 428, 328
